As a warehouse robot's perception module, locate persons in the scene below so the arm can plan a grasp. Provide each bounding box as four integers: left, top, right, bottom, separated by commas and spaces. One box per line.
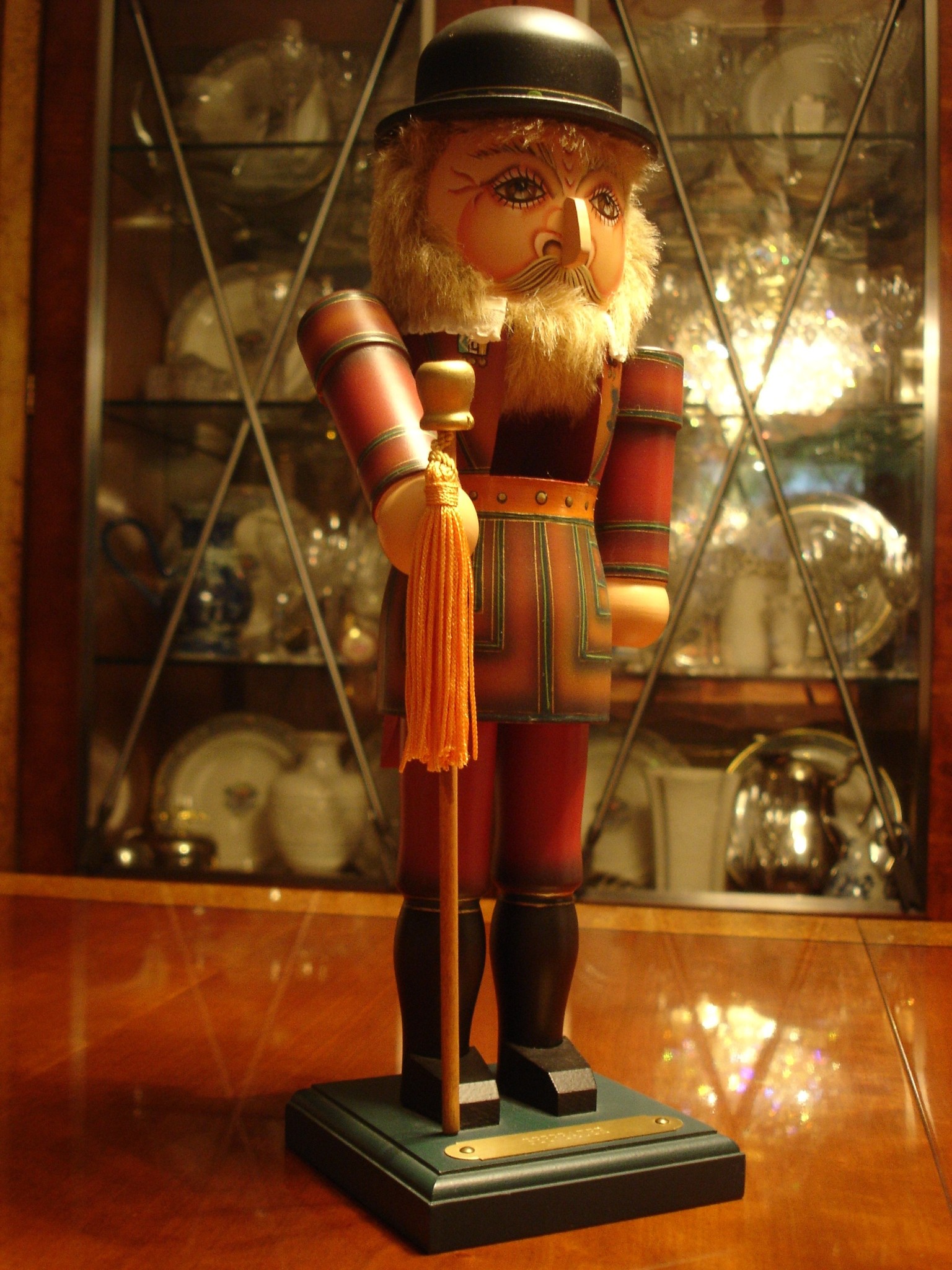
295, 5, 685, 1136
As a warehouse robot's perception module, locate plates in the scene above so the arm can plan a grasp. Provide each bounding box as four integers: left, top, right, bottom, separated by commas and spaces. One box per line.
180, 36, 336, 199
211, 487, 313, 655
165, 261, 318, 402
582, 724, 689, 892
346, 733, 398, 876
149, 712, 299, 877
726, 728, 901, 896
728, 25, 907, 202
743, 492, 907, 663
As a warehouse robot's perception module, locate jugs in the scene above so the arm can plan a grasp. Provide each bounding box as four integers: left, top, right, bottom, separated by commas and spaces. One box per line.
102, 499, 254, 660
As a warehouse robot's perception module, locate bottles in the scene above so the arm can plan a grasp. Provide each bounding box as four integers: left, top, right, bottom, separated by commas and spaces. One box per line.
272, 730, 364, 876
773, 558, 811, 674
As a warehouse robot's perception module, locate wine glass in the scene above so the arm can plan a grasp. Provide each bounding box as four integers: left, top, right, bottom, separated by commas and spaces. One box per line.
651, 21, 713, 132
254, 253, 307, 399
323, 49, 369, 199
829, 8, 918, 159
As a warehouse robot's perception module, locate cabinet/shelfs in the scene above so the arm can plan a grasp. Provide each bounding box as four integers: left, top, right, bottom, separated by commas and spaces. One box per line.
16, 2, 950, 924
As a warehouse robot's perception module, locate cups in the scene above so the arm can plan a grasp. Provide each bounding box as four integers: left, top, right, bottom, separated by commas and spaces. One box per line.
642, 770, 741, 894
